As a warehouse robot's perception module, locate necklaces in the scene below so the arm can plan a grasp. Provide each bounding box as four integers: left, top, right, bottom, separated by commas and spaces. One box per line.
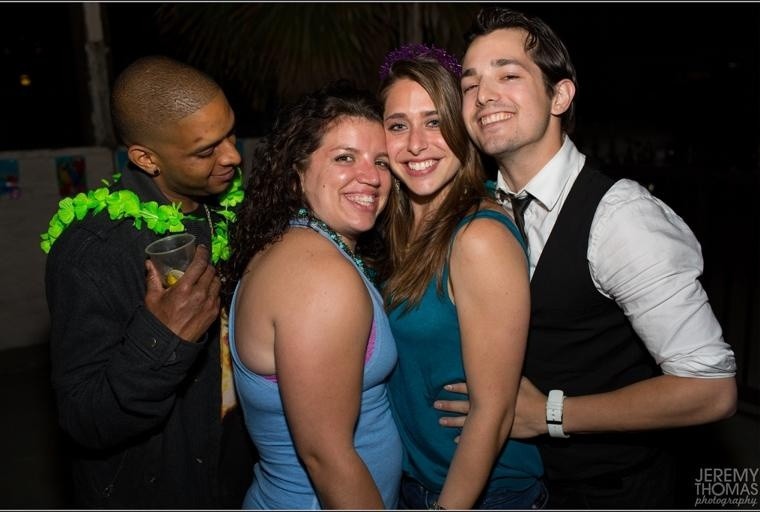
298, 209, 375, 281
203, 202, 227, 284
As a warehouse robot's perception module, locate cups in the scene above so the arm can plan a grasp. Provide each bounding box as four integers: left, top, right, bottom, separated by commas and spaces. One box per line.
145, 233, 196, 290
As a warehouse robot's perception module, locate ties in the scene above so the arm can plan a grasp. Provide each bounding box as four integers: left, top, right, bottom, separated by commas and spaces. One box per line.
508, 195, 535, 242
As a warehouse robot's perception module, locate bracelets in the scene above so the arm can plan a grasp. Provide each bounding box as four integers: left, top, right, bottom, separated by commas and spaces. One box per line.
547, 385, 570, 446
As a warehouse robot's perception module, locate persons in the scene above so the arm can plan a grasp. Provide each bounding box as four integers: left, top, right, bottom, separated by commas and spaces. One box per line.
434, 14, 737, 507
377, 42, 550, 508
43, 56, 243, 508
228, 97, 404, 508
